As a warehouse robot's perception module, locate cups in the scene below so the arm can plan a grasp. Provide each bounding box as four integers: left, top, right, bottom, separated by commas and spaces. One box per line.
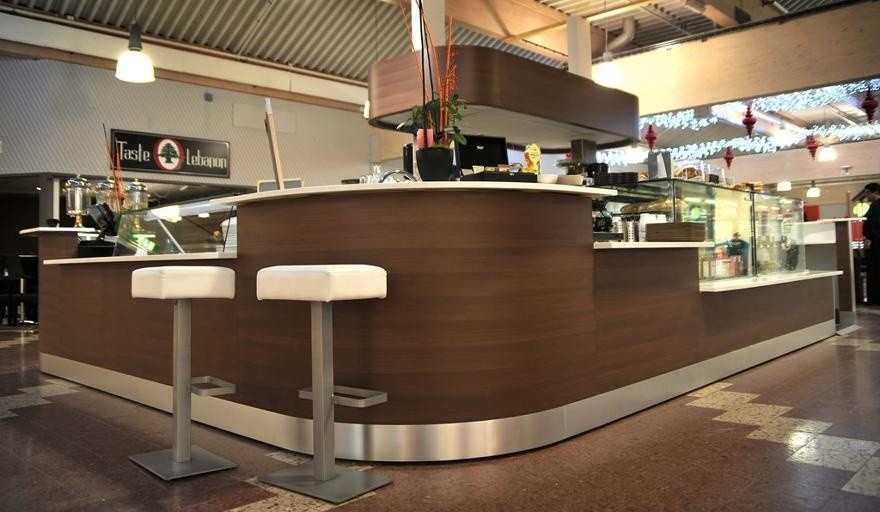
638, 213, 667, 243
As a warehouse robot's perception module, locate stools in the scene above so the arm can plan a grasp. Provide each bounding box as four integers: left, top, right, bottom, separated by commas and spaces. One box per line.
256, 264, 395, 507
130, 265, 235, 485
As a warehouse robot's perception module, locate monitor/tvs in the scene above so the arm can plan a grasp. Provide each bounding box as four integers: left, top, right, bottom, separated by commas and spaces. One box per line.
89, 203, 117, 236
454, 135, 509, 174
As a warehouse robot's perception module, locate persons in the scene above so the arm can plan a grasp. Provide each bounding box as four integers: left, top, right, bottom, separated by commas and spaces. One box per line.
728, 232, 750, 276
863, 182, 880, 306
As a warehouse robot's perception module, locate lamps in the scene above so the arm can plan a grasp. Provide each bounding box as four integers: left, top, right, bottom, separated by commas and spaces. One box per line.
806, 180, 821, 198
817, 143, 838, 162
114, 0, 157, 83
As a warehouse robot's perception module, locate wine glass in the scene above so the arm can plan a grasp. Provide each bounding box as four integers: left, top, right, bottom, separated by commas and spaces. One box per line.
63, 173, 92, 228
93, 176, 122, 213
123, 177, 149, 210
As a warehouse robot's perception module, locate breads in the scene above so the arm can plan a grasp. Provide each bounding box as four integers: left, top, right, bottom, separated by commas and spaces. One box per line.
728, 181, 764, 193
674, 165, 697, 179
619, 198, 689, 214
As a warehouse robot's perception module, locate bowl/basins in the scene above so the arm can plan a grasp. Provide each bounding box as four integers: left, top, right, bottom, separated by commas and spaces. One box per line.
538, 174, 584, 185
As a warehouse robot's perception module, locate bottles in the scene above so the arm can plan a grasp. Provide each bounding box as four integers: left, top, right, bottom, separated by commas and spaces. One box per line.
621, 214, 639, 242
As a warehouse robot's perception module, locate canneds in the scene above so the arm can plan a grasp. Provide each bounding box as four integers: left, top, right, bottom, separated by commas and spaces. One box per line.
699, 255, 715, 280
